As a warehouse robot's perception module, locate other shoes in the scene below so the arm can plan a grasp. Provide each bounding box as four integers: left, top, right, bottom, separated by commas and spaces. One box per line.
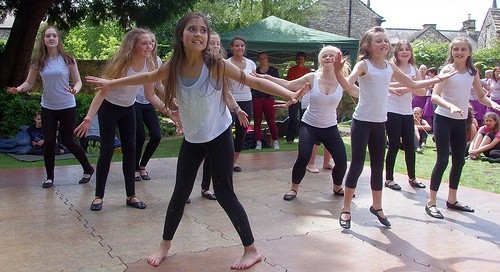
42, 180, 54, 188
369, 206, 391, 226
234, 165, 242, 172
126, 196, 147, 209
134, 176, 141, 181
283, 188, 297, 200
186, 198, 191, 203
425, 203, 444, 219
139, 168, 151, 180
385, 180, 401, 190
79, 168, 94, 184
409, 178, 426, 188
446, 200, 474, 211
322, 162, 335, 169
90, 198, 103, 211
414, 141, 478, 160
333, 188, 355, 198
201, 190, 217, 200
286, 141, 293, 144
339, 212, 352, 229
306, 165, 318, 173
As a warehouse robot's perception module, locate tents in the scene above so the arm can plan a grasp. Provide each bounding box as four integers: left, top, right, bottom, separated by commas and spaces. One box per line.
218, 14, 361, 76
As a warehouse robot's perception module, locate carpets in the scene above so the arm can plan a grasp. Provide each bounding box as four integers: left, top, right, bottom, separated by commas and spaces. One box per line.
6, 150, 99, 162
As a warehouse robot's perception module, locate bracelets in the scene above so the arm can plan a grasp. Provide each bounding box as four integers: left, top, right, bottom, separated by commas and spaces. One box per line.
439, 77, 444, 81
232, 106, 243, 113
165, 108, 173, 117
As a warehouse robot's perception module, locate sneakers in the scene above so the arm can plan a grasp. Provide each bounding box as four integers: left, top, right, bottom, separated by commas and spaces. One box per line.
255, 141, 262, 150
271, 139, 279, 149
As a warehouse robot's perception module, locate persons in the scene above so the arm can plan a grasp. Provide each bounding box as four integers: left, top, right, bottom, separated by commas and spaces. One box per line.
186, 30, 251, 203
6, 25, 95, 188
338, 26, 458, 231
73, 27, 183, 210
252, 52, 279, 151
80, 110, 122, 155
384, 37, 429, 190
272, 60, 350, 173
28, 110, 70, 155
413, 62, 500, 164
425, 37, 500, 219
285, 52, 312, 144
85, 13, 311, 270
127, 29, 179, 182
225, 35, 258, 172
248, 45, 402, 202
